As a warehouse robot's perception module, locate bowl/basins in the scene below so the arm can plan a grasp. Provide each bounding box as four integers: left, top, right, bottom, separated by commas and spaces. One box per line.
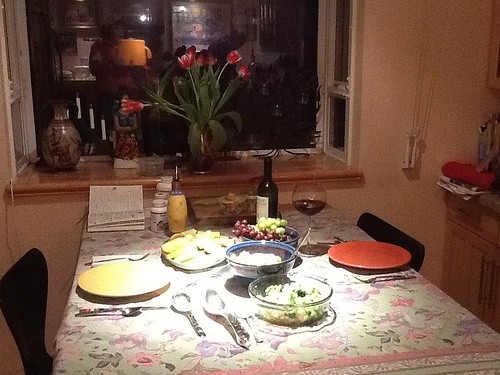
241, 224, 300, 249
248, 273, 334, 327
225, 240, 297, 277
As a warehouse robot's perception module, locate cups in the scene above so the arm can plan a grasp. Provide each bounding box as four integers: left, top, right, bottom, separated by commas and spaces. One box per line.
218, 195, 243, 214
247, 196, 256, 213
139, 156, 164, 177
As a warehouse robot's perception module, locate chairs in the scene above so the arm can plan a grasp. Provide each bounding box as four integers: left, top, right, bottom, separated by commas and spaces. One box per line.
356, 212, 426, 273
0, 247, 53, 375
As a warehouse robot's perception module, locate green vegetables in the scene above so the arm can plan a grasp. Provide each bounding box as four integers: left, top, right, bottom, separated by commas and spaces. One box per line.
281, 289, 320, 322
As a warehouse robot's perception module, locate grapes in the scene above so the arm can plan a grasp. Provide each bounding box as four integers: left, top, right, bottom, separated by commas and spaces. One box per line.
234, 217, 291, 243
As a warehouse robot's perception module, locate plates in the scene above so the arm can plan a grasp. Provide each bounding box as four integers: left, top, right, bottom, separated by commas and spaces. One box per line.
328, 241, 411, 270
78, 261, 169, 297
162, 240, 226, 271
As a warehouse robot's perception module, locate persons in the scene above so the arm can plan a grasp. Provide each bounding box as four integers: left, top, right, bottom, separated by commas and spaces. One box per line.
90, 24, 250, 154
113, 95, 138, 159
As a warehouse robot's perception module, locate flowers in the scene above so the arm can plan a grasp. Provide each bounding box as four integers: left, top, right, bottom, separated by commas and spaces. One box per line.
119, 47, 254, 157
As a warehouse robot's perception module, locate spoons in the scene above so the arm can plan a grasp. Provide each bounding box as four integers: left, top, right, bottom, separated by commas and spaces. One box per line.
75, 307, 142, 317
333, 236, 349, 242
202, 288, 251, 348
171, 293, 206, 338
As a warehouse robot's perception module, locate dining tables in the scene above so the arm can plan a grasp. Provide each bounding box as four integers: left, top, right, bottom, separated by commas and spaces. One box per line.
48, 203, 500, 375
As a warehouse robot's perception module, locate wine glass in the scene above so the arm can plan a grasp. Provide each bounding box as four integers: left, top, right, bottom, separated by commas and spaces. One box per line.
292, 183, 327, 254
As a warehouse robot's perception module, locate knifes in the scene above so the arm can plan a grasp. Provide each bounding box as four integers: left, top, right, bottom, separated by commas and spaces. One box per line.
317, 242, 337, 245
79, 306, 167, 310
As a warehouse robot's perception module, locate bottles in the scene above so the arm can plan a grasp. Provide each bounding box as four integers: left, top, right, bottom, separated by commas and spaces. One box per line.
149, 192, 168, 232
41, 99, 83, 170
155, 175, 174, 205
168, 177, 188, 234
255, 158, 278, 224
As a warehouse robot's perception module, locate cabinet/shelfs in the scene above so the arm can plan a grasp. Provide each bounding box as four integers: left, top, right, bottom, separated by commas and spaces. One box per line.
441, 192, 500, 335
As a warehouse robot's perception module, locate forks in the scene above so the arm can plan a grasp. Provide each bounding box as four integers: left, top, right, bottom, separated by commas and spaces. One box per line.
83, 252, 150, 266
351, 274, 416, 283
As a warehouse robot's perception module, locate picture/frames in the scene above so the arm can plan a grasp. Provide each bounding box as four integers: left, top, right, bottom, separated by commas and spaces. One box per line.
57, 0, 99, 31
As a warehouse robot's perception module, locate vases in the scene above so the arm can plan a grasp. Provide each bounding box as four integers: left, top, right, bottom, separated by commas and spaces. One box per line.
41, 100, 83, 174
189, 152, 217, 175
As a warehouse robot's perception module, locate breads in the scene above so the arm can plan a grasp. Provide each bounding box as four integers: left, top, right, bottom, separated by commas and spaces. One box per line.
162, 229, 233, 268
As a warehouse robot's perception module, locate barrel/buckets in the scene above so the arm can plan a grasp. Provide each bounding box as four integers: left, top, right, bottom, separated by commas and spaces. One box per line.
113, 36, 152, 66
